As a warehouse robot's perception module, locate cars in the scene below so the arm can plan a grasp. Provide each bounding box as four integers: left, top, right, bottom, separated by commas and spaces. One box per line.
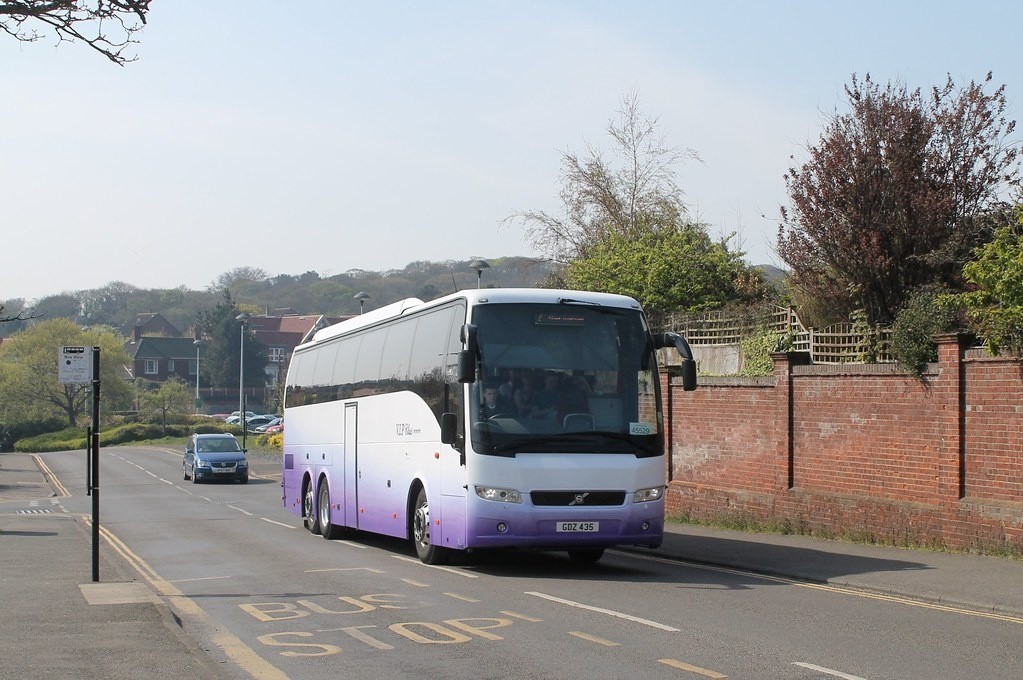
208, 411, 284, 434
182, 433, 249, 484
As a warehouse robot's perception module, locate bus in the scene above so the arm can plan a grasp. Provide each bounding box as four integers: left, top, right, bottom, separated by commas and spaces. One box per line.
282, 286, 698, 566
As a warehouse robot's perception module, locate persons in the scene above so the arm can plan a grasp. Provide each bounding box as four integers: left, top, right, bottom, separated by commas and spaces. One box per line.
482, 385, 500, 417
500, 368, 563, 412
199, 440, 209, 452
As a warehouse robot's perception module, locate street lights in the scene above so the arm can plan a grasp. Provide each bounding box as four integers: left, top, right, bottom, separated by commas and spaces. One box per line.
236, 314, 250, 427
193, 340, 202, 414
470, 260, 490, 290
354, 291, 368, 315
